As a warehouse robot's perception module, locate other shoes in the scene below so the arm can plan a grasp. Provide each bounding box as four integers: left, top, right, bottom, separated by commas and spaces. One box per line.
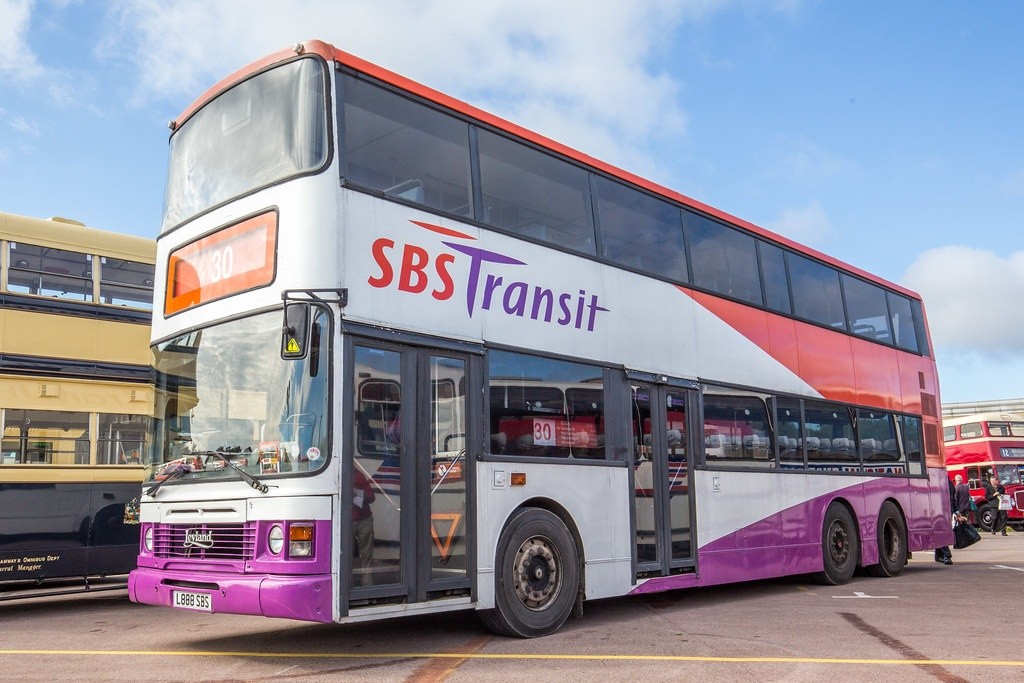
943, 557, 953, 565
1002, 530, 1007, 536
936, 557, 943, 562
992, 527, 995, 535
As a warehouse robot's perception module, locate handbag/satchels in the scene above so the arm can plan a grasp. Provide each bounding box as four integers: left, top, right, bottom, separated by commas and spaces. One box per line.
968, 493, 978, 511
953, 518, 981, 550
996, 494, 1013, 511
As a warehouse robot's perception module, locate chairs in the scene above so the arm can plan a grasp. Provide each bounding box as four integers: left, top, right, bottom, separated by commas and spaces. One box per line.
491, 428, 916, 462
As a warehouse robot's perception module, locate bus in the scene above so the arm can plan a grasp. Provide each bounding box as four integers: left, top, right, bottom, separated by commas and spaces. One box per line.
938, 410, 1024, 531
122, 38, 957, 642
1, 210, 270, 617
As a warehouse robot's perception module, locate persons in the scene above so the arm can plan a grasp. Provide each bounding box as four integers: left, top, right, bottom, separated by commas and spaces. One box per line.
953, 474, 975, 525
986, 476, 1009, 536
935, 473, 962, 565
